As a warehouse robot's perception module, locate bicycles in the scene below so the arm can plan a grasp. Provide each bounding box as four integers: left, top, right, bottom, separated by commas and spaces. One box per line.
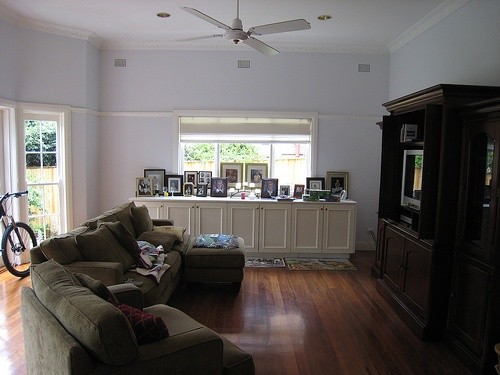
0, 191, 37, 278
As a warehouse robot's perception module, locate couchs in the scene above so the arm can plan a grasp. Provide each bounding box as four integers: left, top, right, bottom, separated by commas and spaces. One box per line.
32, 200, 188, 305
20, 259, 256, 375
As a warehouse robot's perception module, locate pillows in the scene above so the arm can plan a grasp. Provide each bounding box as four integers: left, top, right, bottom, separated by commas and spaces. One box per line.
111, 300, 167, 344
136, 230, 175, 252
153, 224, 186, 242
73, 271, 117, 302
76, 225, 135, 273
131, 204, 154, 232
98, 220, 141, 255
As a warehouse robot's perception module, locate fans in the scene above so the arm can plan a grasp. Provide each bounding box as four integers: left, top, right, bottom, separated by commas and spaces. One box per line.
177, 0, 311, 54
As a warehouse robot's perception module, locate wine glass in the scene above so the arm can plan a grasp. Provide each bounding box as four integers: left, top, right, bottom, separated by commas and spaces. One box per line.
171, 187, 174, 196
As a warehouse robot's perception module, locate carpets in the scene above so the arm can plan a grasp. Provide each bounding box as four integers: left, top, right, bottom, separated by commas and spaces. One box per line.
245, 256, 358, 272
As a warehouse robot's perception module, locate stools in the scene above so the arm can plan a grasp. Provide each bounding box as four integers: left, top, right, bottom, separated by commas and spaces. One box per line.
186, 234, 247, 293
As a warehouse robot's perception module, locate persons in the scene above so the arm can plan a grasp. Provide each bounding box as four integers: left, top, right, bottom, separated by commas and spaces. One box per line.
139, 179, 145, 194
297, 187, 301, 194
170, 182, 177, 191
227, 170, 236, 182
186, 185, 191, 195
335, 179, 340, 190
265, 182, 275, 196
253, 171, 260, 182
213, 179, 225, 193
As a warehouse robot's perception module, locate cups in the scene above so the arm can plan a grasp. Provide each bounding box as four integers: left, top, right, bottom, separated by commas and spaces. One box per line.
162, 187, 167, 192
241, 192, 245, 199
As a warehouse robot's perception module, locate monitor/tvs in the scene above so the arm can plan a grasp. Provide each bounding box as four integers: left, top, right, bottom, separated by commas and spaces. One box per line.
401, 150, 424, 210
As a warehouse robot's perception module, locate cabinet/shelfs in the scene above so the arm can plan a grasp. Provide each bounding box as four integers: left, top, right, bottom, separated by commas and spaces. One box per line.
372, 84, 500, 375
130, 198, 357, 254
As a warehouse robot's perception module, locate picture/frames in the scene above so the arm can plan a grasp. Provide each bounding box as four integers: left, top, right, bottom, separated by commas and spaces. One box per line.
183, 182, 193, 197
165, 175, 183, 195
143, 168, 165, 196
184, 171, 198, 188
197, 163, 348, 198
136, 177, 153, 198
